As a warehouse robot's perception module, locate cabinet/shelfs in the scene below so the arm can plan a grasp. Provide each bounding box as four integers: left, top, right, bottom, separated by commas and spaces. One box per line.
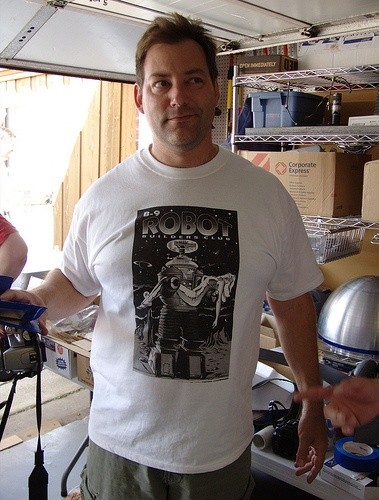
231, 65, 379, 268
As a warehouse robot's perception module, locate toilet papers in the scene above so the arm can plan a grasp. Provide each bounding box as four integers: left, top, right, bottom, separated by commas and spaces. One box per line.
252, 424, 275, 451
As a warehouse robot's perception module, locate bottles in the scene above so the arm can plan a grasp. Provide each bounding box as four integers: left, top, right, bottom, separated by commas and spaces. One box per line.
48, 304, 100, 335
331, 92, 341, 126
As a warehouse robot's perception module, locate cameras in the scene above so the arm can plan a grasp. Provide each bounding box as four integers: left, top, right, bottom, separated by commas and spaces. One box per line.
3, 342, 47, 373
271, 418, 298, 461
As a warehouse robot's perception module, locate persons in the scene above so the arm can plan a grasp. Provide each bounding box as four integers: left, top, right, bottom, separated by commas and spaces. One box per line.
0, 13, 326, 500
294, 377, 379, 434
0, 214, 29, 280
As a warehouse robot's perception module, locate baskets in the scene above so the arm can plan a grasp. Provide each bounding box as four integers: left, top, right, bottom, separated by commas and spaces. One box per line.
304, 224, 365, 264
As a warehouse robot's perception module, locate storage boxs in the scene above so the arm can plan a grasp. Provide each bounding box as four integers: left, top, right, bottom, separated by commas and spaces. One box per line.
248, 93, 326, 128
40, 323, 94, 386
237, 149, 379, 223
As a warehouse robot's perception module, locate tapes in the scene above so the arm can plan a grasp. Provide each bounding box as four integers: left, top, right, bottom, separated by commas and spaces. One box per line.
334, 438, 379, 473
